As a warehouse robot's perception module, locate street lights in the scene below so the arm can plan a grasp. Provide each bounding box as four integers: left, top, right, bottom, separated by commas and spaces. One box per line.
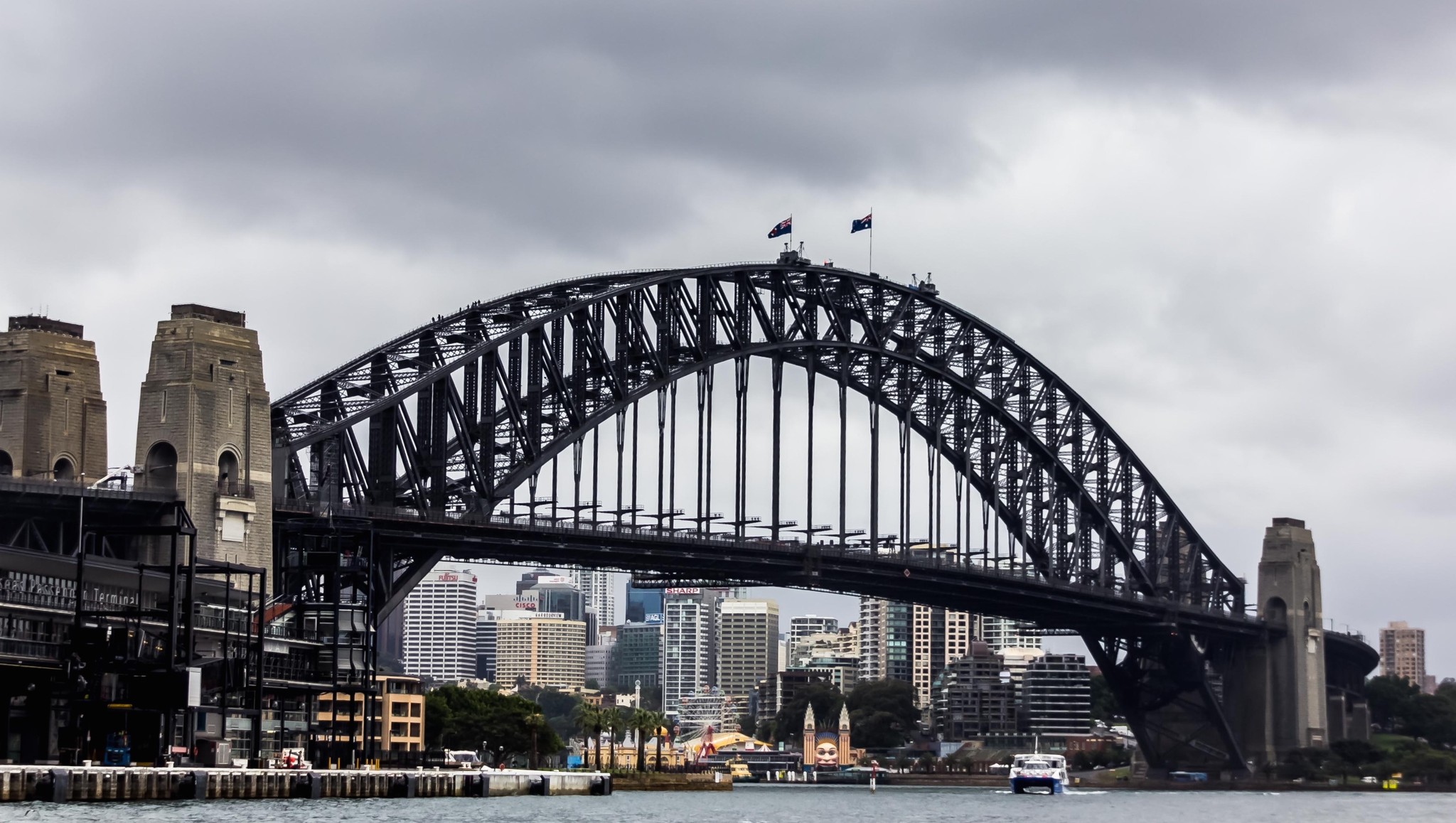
448, 732, 456, 768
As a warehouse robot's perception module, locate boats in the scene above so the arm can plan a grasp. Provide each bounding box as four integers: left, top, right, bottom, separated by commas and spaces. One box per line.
1007, 734, 1070, 795
725, 753, 760, 784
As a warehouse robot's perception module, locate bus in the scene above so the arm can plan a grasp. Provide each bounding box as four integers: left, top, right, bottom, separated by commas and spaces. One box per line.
1167, 771, 1208, 783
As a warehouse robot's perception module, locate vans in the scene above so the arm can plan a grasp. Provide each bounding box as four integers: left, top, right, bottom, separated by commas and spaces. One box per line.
1360, 776, 1378, 784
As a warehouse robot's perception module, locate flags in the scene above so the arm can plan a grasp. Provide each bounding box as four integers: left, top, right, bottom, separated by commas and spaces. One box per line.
850, 213, 871, 233
767, 217, 791, 239
